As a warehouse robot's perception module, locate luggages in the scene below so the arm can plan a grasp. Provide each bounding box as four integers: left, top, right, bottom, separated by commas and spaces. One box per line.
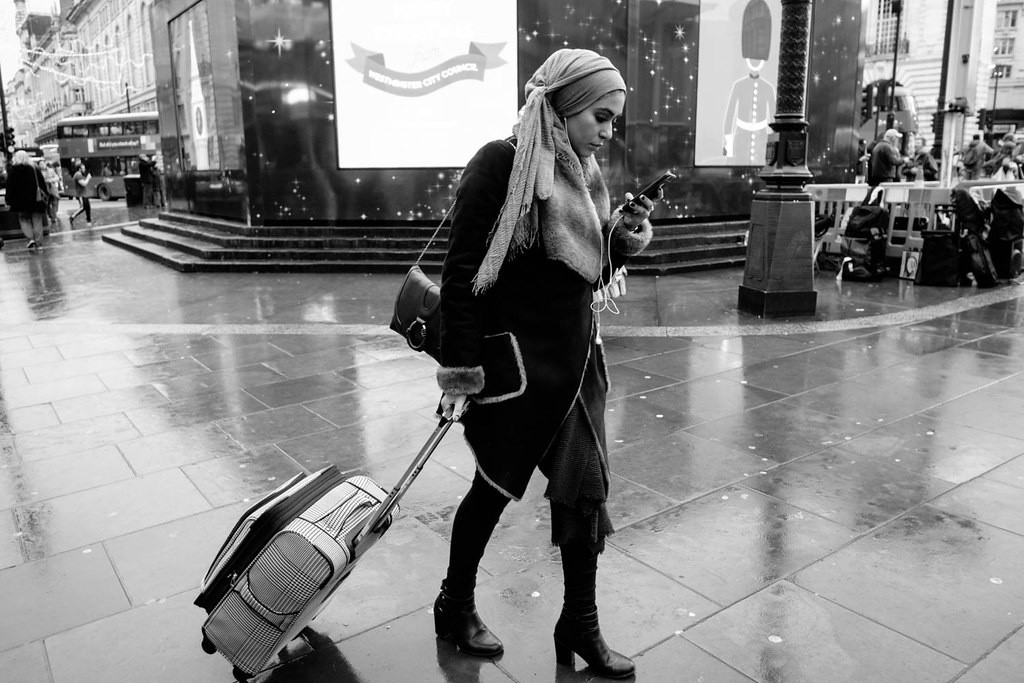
193, 399, 471, 683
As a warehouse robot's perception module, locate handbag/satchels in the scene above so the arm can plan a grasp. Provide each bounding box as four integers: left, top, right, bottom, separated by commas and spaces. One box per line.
390, 139, 518, 366
37, 187, 48, 205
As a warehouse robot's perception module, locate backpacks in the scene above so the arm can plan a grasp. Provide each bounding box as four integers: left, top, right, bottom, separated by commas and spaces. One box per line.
963, 146, 979, 167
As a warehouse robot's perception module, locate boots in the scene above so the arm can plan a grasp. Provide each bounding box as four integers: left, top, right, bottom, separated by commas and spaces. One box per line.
554, 603, 635, 679
433, 578, 502, 657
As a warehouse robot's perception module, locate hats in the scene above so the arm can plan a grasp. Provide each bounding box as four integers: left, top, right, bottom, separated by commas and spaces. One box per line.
973, 134, 979, 139
887, 128, 902, 136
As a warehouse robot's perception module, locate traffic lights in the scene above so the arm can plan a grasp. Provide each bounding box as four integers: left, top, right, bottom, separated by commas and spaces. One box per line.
975, 108, 986, 130
986, 110, 994, 130
4, 127, 15, 154
861, 84, 873, 119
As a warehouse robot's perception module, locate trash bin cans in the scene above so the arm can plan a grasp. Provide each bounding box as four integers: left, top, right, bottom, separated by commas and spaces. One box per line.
124, 174, 144, 206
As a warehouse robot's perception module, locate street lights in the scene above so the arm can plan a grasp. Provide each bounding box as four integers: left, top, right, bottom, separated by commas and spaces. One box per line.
989, 68, 1003, 146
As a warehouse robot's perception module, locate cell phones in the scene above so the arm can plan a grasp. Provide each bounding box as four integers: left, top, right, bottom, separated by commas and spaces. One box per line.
622, 172, 677, 215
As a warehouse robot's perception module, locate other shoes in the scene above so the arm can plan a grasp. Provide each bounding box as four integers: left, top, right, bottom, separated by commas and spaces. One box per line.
27, 241, 36, 248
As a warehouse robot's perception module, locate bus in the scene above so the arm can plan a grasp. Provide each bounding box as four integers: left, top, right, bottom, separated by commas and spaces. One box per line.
11, 143, 70, 197
55, 110, 164, 202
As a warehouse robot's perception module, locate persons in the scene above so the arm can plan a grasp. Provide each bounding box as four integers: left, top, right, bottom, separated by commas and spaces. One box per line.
868, 128, 1020, 186
432, 48, 666, 680
0, 148, 162, 251
